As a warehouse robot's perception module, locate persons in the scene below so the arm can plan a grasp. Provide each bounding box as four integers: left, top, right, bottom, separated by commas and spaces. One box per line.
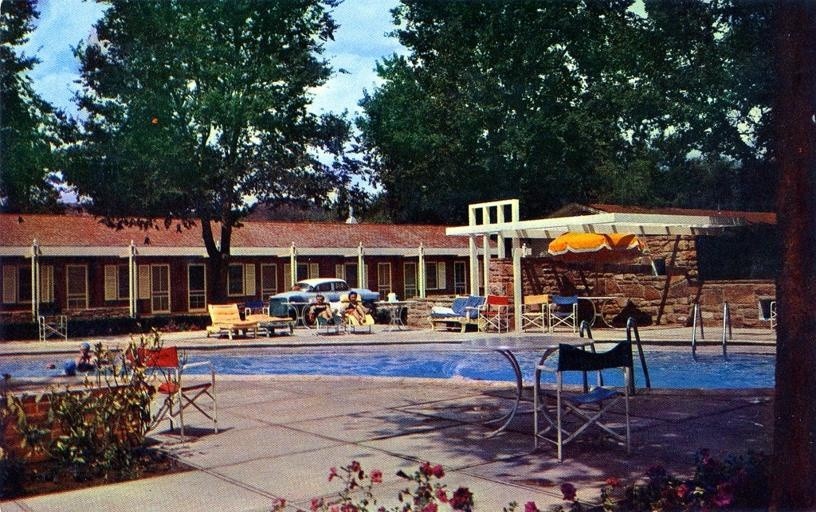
311, 294, 335, 324
63, 343, 99, 376
346, 291, 369, 324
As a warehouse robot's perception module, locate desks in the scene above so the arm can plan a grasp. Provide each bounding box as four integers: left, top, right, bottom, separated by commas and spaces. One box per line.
461, 337, 595, 440
578, 297, 618, 329
281, 302, 313, 332
375, 300, 417, 334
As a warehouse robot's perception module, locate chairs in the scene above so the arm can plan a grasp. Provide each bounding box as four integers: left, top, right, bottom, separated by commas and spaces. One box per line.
38, 303, 68, 342
207, 302, 259, 340
244, 315, 294, 337
340, 294, 374, 335
138, 345, 217, 441
550, 296, 579, 336
535, 341, 631, 464
520, 295, 550, 334
427, 293, 486, 333
477, 295, 509, 334
310, 305, 342, 335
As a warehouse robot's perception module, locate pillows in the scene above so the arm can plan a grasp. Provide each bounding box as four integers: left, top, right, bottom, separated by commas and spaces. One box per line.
453, 296, 485, 318
452, 296, 485, 319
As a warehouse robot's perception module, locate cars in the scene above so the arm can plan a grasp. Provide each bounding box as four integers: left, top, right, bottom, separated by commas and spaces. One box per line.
268, 278, 381, 328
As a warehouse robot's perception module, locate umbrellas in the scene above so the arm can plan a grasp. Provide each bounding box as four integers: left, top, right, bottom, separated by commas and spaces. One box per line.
549, 233, 649, 311
